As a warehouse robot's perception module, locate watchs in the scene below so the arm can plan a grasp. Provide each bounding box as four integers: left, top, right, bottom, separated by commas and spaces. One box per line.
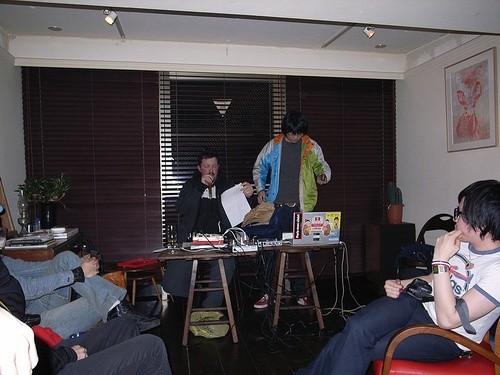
432, 265, 448, 274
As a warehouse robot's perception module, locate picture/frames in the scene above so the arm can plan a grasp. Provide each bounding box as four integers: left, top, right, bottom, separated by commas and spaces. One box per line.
444, 47, 496, 153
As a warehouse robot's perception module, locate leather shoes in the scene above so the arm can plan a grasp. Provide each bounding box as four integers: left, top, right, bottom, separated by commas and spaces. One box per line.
107, 299, 161, 332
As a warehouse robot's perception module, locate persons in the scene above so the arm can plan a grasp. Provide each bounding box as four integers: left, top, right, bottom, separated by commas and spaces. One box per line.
0, 206, 172, 375
312, 179, 500, 375
163, 152, 253, 309
253, 112, 331, 308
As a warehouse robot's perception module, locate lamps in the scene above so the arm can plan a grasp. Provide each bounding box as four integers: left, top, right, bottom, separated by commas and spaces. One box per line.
363, 26, 376, 38
103, 9, 117, 25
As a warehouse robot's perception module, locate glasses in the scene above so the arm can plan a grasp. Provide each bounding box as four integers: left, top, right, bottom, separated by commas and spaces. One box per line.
453, 208, 465, 221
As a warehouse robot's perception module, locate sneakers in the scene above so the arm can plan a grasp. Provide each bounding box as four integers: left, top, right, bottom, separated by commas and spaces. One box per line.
254, 294, 276, 308
297, 296, 309, 306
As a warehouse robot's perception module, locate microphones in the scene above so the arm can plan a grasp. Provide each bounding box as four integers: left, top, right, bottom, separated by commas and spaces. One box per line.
209, 175, 214, 197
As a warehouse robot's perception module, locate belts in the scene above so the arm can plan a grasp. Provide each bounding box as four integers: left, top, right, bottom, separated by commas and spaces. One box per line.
274, 201, 300, 207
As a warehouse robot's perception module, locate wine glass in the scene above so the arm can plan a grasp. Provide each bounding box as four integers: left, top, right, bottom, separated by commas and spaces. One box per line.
168, 225, 177, 255
205, 178, 215, 202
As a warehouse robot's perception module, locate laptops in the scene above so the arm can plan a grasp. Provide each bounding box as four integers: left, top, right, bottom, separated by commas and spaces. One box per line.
282, 211, 341, 246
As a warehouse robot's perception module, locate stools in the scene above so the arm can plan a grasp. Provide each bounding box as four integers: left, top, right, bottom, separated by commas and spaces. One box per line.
116, 259, 167, 330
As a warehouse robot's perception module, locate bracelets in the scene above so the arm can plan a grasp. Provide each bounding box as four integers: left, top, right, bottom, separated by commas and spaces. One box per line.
431, 260, 469, 281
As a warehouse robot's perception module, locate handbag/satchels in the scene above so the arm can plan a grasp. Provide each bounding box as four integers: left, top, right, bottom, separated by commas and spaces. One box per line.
402, 278, 434, 303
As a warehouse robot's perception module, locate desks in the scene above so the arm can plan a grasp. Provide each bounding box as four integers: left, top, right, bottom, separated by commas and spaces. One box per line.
364, 223, 416, 272
1, 229, 82, 263
159, 240, 345, 344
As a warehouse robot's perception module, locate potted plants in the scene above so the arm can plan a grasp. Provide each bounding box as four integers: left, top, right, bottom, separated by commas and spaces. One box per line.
13, 169, 76, 229
386, 181, 405, 224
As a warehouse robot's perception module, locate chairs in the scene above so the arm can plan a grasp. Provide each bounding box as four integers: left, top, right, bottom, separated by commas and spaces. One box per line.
370, 315, 500, 375
380, 212, 455, 280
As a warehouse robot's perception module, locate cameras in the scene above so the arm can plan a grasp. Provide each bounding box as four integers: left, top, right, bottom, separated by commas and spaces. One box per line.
405, 279, 435, 300
